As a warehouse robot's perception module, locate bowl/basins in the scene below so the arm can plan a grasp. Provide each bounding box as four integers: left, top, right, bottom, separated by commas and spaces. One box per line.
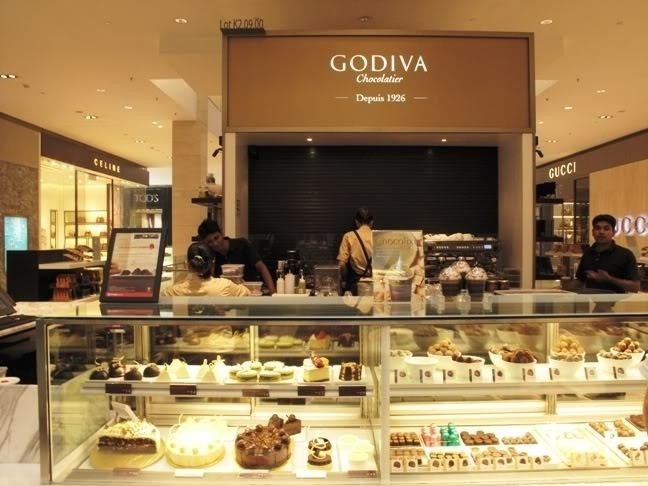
549, 356, 582, 375
415, 330, 455, 351
458, 329, 493, 349
495, 326, 517, 343
452, 355, 484, 380
549, 350, 586, 358
402, 356, 437, 376
392, 328, 413, 344
428, 351, 462, 370
568, 330, 605, 347
610, 346, 645, 368
500, 358, 537, 378
516, 330, 543, 346
601, 330, 642, 351
390, 350, 412, 370
596, 354, 631, 374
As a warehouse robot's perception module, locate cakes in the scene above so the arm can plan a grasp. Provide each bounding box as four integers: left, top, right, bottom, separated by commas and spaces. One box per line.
107, 268, 156, 293
92, 354, 363, 468
391, 321, 626, 351
439, 259, 487, 295
391, 339, 648, 471
149, 325, 355, 352
387, 256, 414, 301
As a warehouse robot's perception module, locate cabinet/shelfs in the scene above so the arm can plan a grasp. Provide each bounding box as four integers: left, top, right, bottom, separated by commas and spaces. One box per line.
36, 293, 648, 485
6, 249, 64, 301
536, 198, 564, 280
64, 210, 108, 252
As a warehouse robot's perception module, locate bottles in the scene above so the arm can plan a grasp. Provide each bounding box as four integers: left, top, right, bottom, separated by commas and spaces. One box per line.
284, 270, 295, 294
296, 276, 306, 294
277, 275, 285, 294
53, 270, 101, 300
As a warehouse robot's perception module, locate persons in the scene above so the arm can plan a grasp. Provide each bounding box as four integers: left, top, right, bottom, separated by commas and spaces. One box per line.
159, 242, 251, 336
575, 215, 640, 293
197, 219, 277, 294
337, 208, 383, 296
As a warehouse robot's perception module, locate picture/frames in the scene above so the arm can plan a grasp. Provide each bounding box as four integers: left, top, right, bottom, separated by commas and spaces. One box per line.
99, 229, 168, 302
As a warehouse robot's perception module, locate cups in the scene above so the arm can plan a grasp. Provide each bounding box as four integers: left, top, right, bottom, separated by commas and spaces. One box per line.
136, 212, 142, 228
465, 278, 485, 301
440, 272, 468, 303
427, 232, 475, 241
147, 212, 154, 228
277, 261, 285, 270
387, 265, 414, 302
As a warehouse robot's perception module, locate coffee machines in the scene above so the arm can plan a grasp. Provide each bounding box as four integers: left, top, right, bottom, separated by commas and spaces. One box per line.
284, 250, 300, 274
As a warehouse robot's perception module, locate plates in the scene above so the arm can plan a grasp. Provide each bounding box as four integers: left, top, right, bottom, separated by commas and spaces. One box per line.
487, 350, 497, 365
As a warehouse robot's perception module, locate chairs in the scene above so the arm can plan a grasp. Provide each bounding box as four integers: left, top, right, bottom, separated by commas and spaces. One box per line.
536, 256, 560, 280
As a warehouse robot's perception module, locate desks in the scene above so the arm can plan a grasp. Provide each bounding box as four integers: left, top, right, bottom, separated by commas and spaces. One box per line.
39, 257, 107, 302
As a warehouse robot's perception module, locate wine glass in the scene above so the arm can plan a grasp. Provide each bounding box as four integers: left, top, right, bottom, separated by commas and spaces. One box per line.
84, 232, 92, 247
